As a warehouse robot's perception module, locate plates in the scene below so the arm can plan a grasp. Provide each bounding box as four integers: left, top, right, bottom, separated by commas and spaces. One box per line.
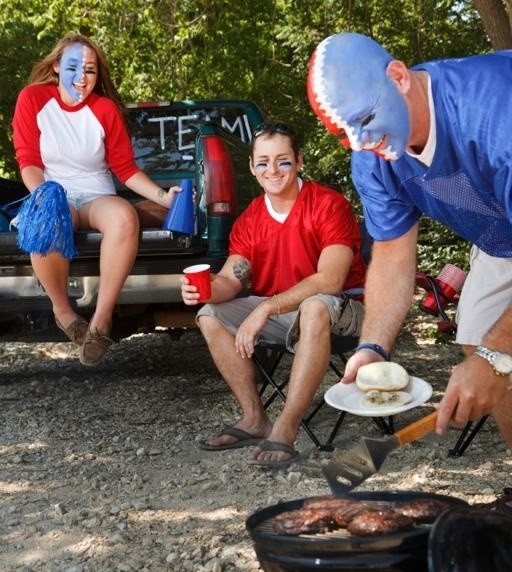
323, 375, 433, 419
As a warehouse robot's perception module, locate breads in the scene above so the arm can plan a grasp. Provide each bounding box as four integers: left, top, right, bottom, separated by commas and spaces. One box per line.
357, 362, 411, 409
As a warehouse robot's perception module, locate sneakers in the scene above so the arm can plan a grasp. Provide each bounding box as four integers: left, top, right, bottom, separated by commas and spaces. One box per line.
54, 314, 88, 344
80, 314, 110, 367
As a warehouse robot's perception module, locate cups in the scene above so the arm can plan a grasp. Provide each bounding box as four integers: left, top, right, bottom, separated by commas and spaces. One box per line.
183, 262, 212, 302
418, 263, 466, 317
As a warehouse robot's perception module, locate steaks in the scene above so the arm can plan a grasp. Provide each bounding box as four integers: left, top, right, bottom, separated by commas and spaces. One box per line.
274, 493, 446, 535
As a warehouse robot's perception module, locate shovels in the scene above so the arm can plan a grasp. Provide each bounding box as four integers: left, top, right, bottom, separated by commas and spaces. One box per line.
321, 411, 437, 498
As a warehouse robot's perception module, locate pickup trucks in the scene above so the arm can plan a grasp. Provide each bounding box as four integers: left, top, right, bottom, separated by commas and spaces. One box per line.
0, 96, 270, 343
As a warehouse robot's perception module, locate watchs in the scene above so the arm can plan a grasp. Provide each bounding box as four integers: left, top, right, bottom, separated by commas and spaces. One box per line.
472, 346, 512, 376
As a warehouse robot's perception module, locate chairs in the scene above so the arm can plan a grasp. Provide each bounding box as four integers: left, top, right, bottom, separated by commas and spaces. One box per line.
411, 269, 498, 458
243, 220, 397, 457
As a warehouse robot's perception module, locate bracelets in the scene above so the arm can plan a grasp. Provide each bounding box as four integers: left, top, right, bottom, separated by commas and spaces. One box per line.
355, 343, 391, 361
273, 294, 281, 315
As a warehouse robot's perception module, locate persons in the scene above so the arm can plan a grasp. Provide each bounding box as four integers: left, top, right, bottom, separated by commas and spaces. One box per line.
181, 121, 366, 466
306, 32, 512, 453
13, 34, 197, 366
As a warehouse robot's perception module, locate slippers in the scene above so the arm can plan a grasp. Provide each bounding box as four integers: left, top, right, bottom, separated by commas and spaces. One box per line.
197, 424, 266, 452
247, 433, 301, 467
